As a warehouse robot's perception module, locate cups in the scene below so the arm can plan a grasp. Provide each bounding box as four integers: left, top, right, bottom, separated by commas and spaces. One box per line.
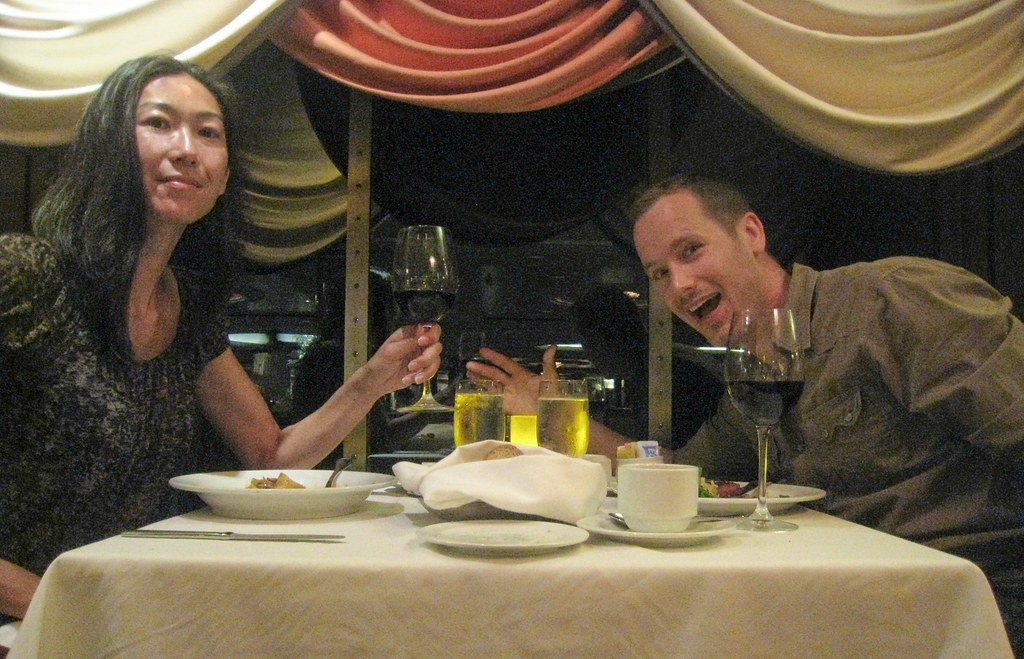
456, 379, 506, 447
534, 380, 590, 460
617, 464, 700, 533
616, 457, 666, 482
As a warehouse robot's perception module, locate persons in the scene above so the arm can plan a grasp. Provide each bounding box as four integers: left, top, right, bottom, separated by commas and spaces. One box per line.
287, 271, 463, 474
0, 54, 444, 659
570, 289, 726, 450
465, 174, 1024, 659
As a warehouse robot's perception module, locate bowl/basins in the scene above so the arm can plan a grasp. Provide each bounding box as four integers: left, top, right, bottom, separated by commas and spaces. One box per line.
169, 469, 399, 520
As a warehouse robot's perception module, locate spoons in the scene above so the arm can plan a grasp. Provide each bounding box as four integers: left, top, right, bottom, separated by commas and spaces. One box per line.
608, 511, 772, 528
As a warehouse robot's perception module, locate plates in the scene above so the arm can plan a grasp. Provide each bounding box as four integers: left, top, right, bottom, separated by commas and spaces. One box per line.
697, 480, 826, 517
577, 514, 736, 546
416, 520, 590, 558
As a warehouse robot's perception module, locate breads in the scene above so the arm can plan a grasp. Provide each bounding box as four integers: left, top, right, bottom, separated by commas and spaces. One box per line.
485, 444, 524, 460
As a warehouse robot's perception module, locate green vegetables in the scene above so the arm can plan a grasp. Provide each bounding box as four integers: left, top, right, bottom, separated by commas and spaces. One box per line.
698, 484, 715, 497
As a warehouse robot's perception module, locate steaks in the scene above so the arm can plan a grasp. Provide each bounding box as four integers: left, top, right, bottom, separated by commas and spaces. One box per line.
714, 482, 742, 498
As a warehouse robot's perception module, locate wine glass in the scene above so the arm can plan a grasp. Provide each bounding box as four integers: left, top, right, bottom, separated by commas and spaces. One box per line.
390, 226, 459, 414
723, 308, 806, 533
454, 330, 486, 380
583, 375, 607, 423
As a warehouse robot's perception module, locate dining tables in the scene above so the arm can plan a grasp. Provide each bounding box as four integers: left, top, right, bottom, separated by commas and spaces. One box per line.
5, 481, 1018, 659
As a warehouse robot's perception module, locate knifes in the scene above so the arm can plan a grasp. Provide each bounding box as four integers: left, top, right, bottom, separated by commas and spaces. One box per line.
120, 529, 345, 541
